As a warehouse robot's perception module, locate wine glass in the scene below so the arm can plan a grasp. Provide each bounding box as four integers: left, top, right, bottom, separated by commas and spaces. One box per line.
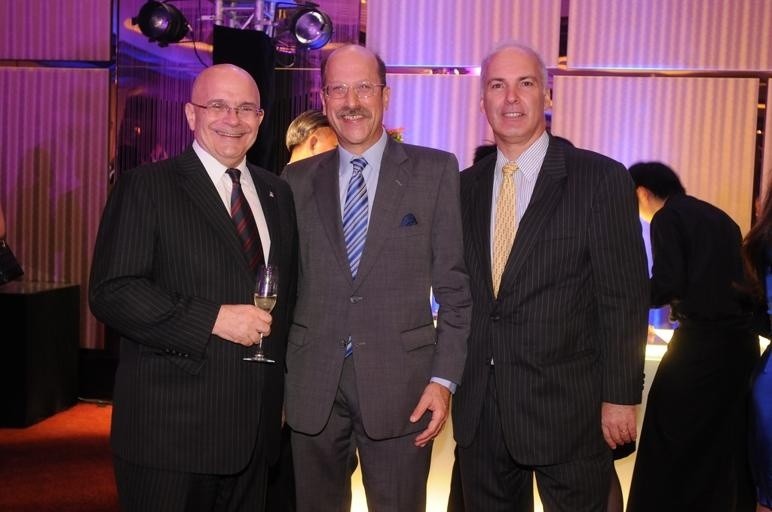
239, 265, 280, 364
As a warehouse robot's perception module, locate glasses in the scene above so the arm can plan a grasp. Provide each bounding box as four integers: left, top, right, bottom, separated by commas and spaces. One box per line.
322, 81, 386, 98
190, 101, 261, 121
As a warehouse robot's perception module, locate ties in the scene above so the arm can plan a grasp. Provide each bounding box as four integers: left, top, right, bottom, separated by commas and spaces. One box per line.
343, 158, 369, 357
492, 162, 519, 299
227, 167, 265, 278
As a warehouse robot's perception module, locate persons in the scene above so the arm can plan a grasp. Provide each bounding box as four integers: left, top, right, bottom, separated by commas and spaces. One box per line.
445, 43, 651, 512
280, 44, 474, 512
88, 64, 299, 512
270, 110, 339, 512
625, 161, 769, 512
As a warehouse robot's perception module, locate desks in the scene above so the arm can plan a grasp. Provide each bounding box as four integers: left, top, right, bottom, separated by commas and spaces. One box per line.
0, 279, 80, 426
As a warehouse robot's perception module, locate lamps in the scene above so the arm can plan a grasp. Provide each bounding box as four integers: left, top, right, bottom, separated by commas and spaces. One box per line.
129, 0, 333, 172
